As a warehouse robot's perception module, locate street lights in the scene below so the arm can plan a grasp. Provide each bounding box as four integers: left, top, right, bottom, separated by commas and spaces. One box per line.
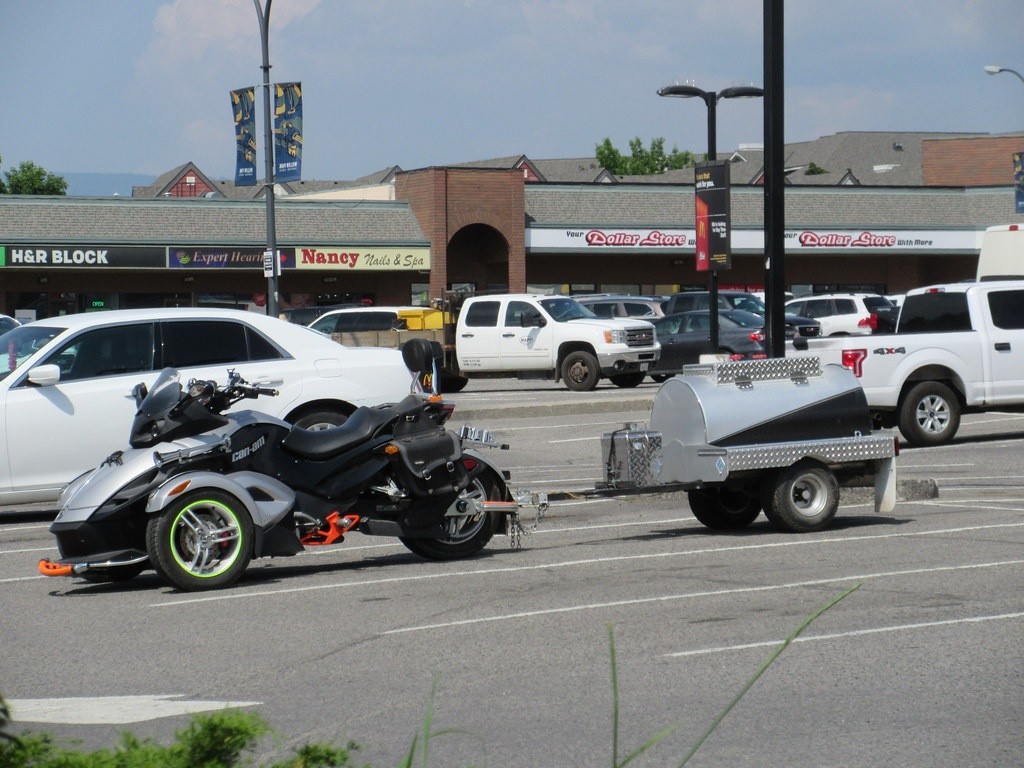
656, 83, 767, 356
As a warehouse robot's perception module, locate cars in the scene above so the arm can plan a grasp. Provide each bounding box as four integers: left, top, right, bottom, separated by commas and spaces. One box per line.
0, 303, 432, 510
630, 307, 773, 375
661, 288, 819, 345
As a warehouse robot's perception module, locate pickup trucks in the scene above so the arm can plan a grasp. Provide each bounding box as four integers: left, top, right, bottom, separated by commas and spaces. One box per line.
782, 275, 1024, 444
325, 293, 660, 393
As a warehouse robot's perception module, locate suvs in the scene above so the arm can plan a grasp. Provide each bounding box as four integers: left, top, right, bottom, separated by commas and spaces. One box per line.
775, 294, 900, 338
305, 306, 465, 391
565, 294, 668, 326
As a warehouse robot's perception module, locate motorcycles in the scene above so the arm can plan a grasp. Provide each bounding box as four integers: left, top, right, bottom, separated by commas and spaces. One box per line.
48, 334, 515, 592
